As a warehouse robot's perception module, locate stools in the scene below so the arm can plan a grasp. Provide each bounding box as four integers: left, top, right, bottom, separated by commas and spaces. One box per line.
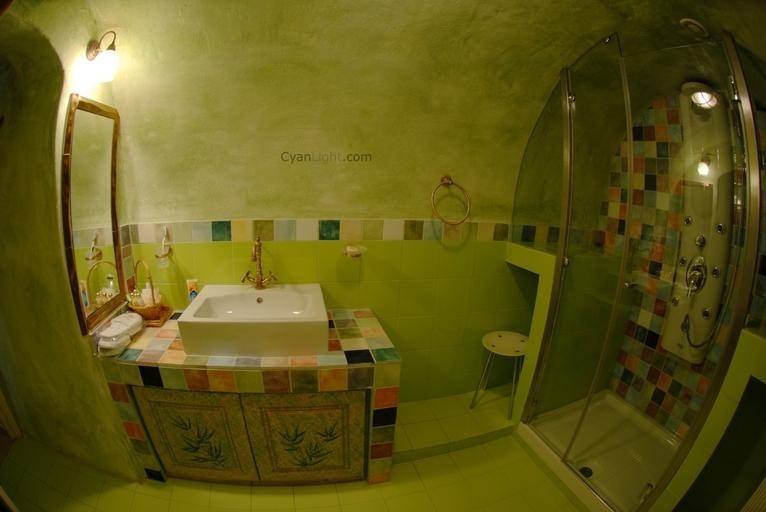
469, 331, 530, 421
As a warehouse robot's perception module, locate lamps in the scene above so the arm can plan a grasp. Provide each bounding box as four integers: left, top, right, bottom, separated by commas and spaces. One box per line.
86, 30, 118, 83
698, 148, 721, 176
681, 81, 724, 112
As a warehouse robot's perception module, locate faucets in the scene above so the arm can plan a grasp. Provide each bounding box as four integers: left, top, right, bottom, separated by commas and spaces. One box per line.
252, 242, 263, 288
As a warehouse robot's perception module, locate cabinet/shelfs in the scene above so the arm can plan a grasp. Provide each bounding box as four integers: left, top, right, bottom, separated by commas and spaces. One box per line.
130, 385, 366, 485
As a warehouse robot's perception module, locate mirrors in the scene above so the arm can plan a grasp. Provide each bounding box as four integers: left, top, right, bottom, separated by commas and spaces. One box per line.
63, 95, 129, 337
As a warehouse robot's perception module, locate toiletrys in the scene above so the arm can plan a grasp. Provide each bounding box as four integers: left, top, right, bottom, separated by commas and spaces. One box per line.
128, 282, 160, 307
94, 286, 119, 309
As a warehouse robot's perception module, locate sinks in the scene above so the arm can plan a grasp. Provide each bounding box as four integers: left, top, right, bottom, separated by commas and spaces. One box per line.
176, 283, 329, 355
